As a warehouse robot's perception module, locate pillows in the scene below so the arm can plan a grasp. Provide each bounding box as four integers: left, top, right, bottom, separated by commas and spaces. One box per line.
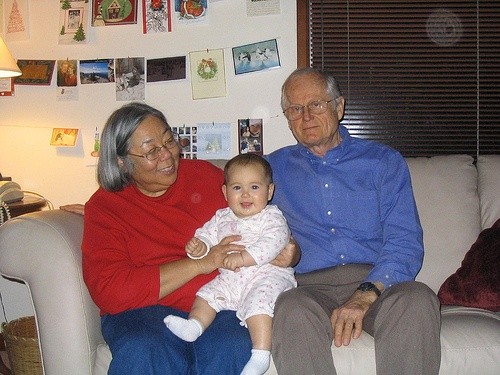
437, 218, 500, 312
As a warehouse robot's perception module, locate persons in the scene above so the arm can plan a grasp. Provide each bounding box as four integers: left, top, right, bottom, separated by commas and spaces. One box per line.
264, 67, 442, 375
238, 120, 263, 155
82, 101, 301, 375
161, 154, 296, 375
169, 125, 197, 160
58, 62, 77, 85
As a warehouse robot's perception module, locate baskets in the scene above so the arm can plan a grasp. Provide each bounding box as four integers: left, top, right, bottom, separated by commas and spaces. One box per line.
0, 315, 44, 375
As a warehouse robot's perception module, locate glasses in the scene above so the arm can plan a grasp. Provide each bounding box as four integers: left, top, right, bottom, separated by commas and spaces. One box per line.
282, 93, 342, 121
123, 131, 180, 162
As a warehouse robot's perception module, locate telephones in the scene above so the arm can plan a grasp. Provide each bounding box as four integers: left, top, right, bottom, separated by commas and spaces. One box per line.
0, 180, 24, 203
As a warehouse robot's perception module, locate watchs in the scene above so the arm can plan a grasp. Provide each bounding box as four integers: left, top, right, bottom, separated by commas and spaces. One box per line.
356, 282, 381, 297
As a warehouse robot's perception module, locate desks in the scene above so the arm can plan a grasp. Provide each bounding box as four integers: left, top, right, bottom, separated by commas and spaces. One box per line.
0, 194, 46, 226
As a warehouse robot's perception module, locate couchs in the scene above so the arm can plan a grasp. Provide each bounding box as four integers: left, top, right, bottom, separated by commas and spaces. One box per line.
0, 153, 500, 375
